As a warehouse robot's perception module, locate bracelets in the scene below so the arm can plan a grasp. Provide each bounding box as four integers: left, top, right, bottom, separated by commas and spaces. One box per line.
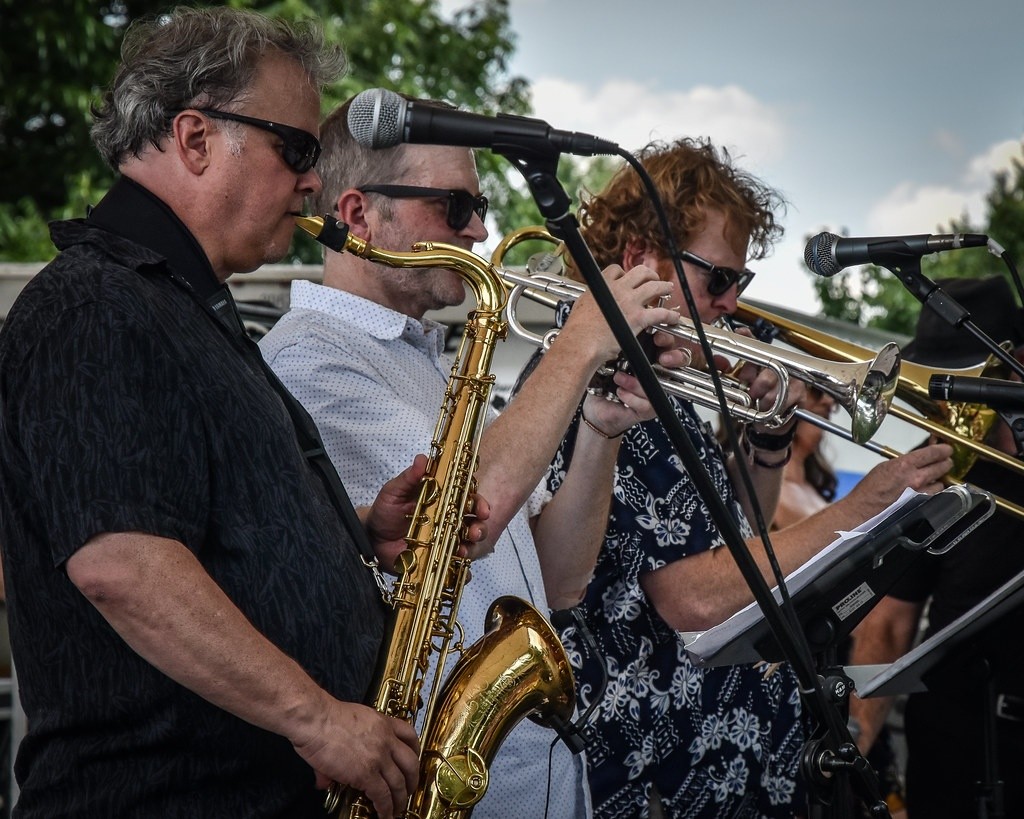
580, 403, 625, 441
743, 442, 792, 470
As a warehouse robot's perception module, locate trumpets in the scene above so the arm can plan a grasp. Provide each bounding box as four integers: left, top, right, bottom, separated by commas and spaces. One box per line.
490, 265, 902, 445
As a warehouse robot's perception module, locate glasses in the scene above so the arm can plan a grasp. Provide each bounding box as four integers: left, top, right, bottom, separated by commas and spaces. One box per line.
163, 109, 323, 173
661, 243, 755, 299
333, 181, 493, 230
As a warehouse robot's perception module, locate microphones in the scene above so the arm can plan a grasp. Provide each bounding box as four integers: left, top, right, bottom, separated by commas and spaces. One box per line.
804, 231, 991, 276
345, 87, 620, 161
929, 374, 1024, 410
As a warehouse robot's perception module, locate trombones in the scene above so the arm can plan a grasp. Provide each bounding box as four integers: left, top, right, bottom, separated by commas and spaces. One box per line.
487, 222, 1022, 520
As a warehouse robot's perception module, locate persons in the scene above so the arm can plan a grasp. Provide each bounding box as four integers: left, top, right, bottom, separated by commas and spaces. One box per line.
769, 370, 905, 818
257, 90, 694, 819
1, 10, 490, 819
505, 140, 952, 819
840, 345, 1024, 819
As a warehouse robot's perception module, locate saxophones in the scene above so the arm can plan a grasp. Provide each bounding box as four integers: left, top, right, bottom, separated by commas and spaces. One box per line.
280, 210, 577, 819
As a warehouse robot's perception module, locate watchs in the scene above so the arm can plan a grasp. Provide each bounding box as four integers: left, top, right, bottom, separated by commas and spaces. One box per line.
743, 422, 797, 451
